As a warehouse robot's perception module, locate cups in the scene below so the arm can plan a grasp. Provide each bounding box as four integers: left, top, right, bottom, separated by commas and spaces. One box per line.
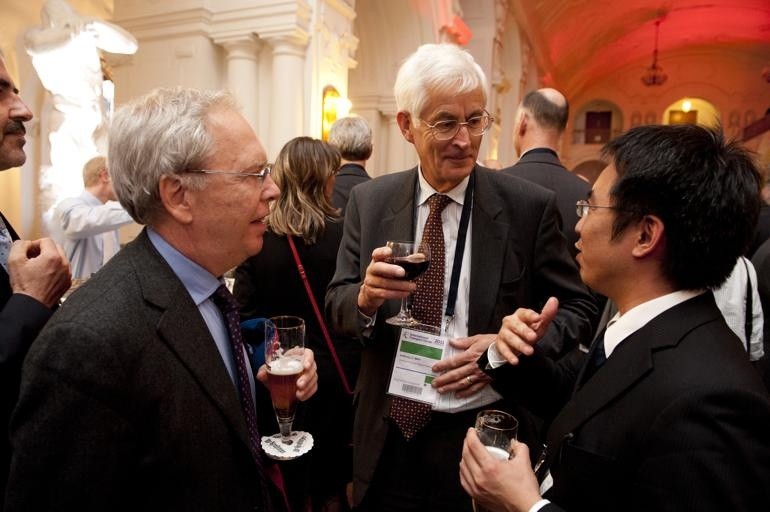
472, 408, 517, 511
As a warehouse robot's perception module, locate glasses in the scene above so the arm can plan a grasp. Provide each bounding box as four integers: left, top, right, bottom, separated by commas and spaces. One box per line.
575, 200, 613, 217
418, 108, 494, 141
184, 163, 273, 189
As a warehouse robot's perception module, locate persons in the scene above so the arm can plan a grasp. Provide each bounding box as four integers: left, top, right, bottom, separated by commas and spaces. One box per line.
1, 41, 770, 510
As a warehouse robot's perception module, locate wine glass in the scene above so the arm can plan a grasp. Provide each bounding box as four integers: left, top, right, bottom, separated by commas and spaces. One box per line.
385, 241, 432, 327
263, 314, 310, 460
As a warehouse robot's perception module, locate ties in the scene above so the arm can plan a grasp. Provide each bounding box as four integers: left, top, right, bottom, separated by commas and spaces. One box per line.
209, 282, 263, 473
388, 193, 451, 442
574, 325, 606, 396
0, 216, 13, 275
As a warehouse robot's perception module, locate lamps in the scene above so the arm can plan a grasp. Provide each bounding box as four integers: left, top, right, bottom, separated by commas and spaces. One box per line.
641, 17, 667, 87
